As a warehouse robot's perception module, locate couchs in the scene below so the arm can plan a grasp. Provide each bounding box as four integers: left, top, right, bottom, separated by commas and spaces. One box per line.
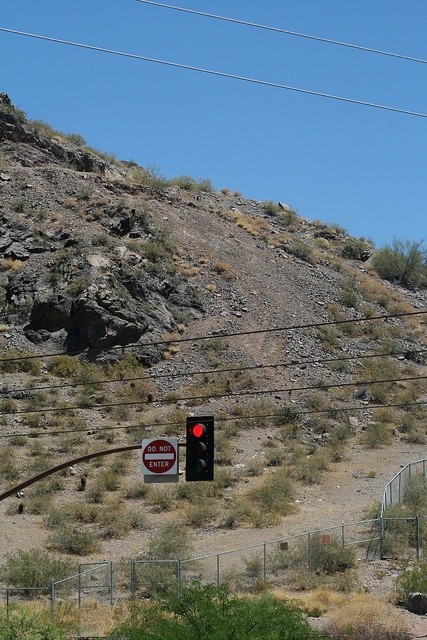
186, 416, 214, 481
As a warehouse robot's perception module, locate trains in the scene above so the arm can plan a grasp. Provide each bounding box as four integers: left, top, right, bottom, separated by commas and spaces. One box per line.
141, 439, 178, 475
